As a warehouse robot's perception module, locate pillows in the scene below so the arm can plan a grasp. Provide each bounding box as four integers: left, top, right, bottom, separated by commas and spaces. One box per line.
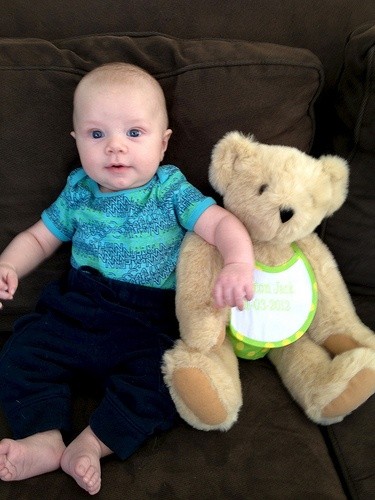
0, 31, 326, 332
316, 20, 375, 298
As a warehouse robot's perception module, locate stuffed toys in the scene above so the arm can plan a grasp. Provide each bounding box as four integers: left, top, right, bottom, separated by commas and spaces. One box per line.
162, 130, 375, 430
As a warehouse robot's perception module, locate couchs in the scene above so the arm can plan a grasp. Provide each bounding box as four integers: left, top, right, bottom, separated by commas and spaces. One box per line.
0, 0, 375, 500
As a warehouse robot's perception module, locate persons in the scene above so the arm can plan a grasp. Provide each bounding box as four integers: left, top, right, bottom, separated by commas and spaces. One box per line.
0, 63, 255, 494
